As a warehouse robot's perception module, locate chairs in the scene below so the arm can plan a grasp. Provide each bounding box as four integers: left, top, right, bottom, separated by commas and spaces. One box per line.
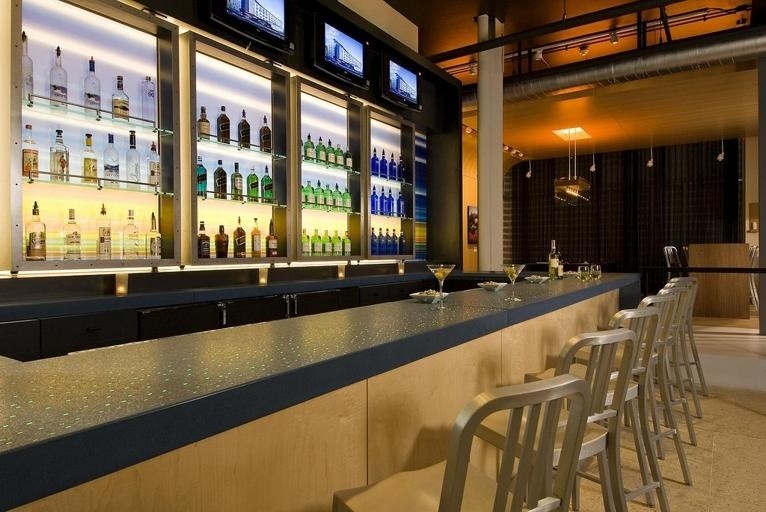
645, 287, 703, 461
524, 306, 672, 512
577, 296, 695, 508
331, 371, 595, 512
662, 239, 760, 321
655, 274, 711, 433
471, 327, 640, 512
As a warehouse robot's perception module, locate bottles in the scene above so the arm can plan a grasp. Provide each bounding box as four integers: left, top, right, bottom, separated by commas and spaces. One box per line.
196, 103, 279, 262
548, 240, 564, 281
17, 30, 161, 264
299, 134, 357, 259
369, 142, 411, 257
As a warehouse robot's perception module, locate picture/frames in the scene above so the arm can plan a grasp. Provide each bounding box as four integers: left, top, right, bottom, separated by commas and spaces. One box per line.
467, 205, 478, 245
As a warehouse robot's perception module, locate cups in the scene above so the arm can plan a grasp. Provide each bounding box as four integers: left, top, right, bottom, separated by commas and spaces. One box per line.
590, 265, 602, 280
576, 265, 589, 281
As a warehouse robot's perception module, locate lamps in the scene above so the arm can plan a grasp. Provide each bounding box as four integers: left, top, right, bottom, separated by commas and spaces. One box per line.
464, 125, 525, 160
716, 138, 724, 161
551, 125, 592, 211
589, 153, 595, 172
525, 160, 531, 178
439, 9, 726, 75
645, 144, 654, 167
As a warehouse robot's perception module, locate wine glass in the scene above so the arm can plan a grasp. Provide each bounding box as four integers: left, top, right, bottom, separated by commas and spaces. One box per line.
425, 263, 455, 311
500, 263, 526, 303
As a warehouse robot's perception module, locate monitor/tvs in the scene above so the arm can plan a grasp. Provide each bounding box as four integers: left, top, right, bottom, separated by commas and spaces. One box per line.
197, 0, 296, 57
373, 49, 424, 114
305, 11, 370, 92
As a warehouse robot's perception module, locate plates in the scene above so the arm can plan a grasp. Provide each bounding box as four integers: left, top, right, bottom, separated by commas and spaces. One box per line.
476, 281, 507, 292
523, 276, 550, 284
409, 291, 448, 303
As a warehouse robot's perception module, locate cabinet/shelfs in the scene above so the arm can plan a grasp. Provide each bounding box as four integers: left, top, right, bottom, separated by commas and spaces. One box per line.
393, 280, 425, 303
19, 89, 177, 264
1, 317, 43, 362
140, 300, 227, 341
39, 308, 138, 362
425, 279, 508, 294
300, 146, 360, 257
352, 281, 392, 308
364, 157, 412, 260
229, 285, 351, 328
195, 117, 292, 263
